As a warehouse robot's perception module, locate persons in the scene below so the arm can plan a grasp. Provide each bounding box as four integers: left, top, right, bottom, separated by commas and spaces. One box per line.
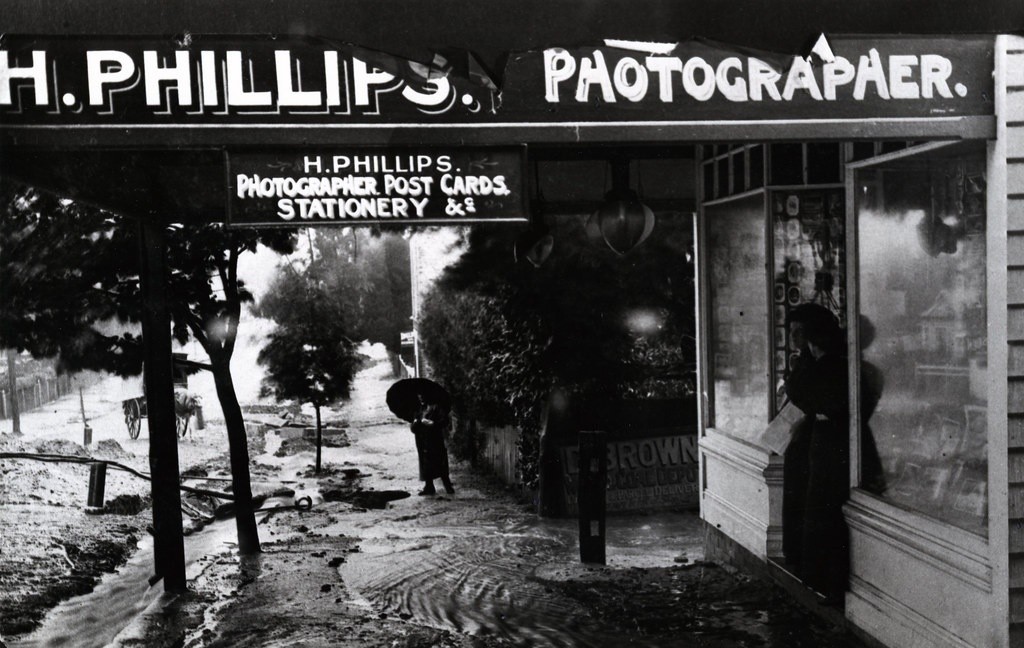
411, 393, 455, 495
788, 303, 849, 587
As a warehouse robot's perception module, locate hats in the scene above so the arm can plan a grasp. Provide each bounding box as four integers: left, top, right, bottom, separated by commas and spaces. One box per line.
786, 303, 844, 338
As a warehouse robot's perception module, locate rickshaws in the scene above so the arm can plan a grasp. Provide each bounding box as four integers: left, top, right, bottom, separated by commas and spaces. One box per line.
122, 391, 194, 439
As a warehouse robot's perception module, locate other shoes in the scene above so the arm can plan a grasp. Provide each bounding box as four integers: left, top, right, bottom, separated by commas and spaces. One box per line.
418, 490, 435, 495
446, 488, 454, 494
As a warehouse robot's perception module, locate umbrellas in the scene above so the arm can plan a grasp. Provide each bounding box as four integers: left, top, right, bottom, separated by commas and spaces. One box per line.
387, 377, 451, 423
